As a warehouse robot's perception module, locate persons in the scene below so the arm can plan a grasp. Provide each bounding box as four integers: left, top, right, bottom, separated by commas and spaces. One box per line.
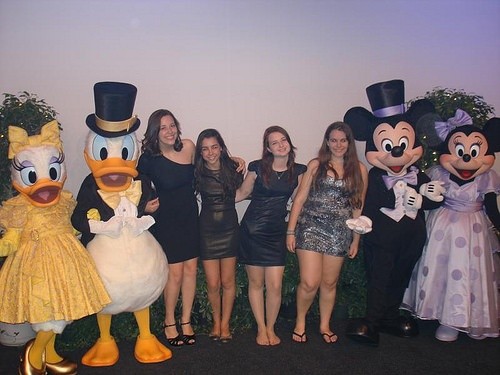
193, 129, 252, 343
286, 122, 368, 344
137, 108, 246, 347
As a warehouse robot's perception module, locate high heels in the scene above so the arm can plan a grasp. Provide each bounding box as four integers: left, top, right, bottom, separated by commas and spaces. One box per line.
179, 318, 197, 345
163, 323, 184, 345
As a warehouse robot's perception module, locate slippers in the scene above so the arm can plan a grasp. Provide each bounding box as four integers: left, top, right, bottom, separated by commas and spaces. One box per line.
320, 333, 339, 343
292, 322, 308, 343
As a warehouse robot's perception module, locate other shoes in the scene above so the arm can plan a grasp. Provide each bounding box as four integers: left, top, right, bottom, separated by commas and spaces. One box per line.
206, 332, 232, 343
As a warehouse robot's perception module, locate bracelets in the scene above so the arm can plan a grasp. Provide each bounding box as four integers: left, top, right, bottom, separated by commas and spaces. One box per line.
235, 126, 308, 346
286, 230, 295, 235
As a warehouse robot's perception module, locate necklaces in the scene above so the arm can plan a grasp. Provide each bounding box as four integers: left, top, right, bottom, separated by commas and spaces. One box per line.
275, 171, 288, 180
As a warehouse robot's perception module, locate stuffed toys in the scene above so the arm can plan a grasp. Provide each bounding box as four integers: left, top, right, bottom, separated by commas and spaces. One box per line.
344, 79, 447, 347
70, 81, 173, 366
399, 108, 500, 341
0, 120, 112, 375
